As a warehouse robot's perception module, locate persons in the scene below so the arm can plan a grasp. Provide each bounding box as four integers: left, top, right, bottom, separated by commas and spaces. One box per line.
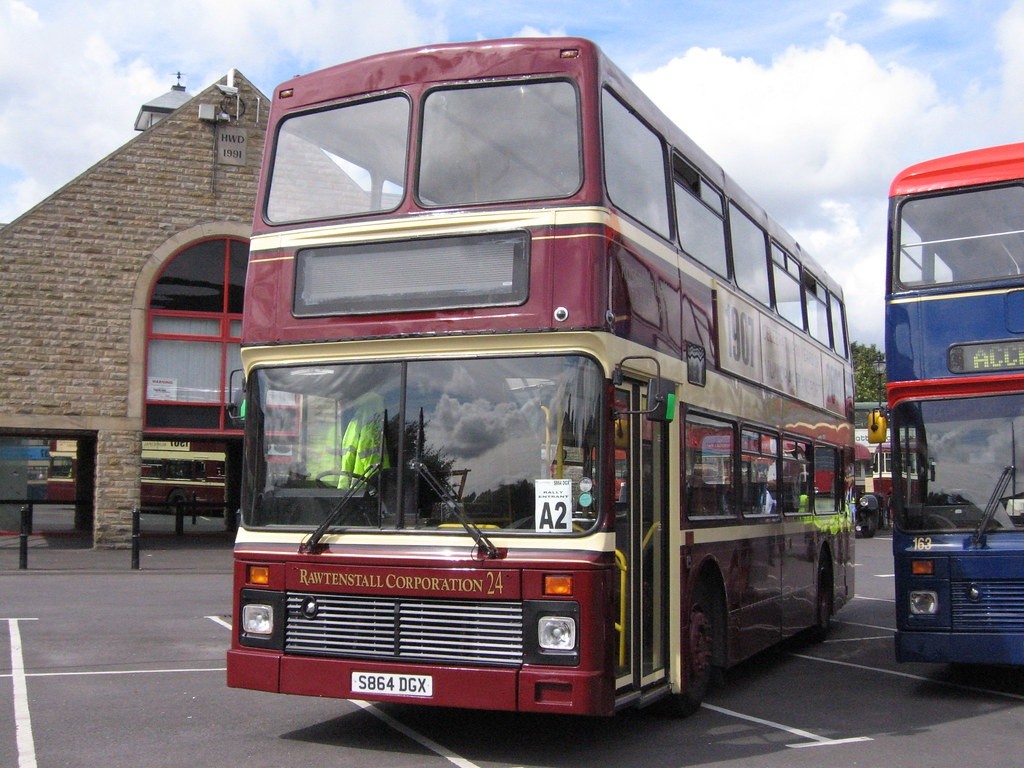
335, 365, 390, 490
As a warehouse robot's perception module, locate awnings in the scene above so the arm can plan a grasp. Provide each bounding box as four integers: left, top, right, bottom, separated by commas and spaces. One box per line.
875, 444, 917, 453
855, 443, 871, 460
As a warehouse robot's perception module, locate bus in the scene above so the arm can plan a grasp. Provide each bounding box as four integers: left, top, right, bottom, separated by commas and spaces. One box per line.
4, 447, 76, 500
225, 39, 855, 716
810, 402, 916, 538
142, 449, 225, 517
868, 146, 1023, 669
